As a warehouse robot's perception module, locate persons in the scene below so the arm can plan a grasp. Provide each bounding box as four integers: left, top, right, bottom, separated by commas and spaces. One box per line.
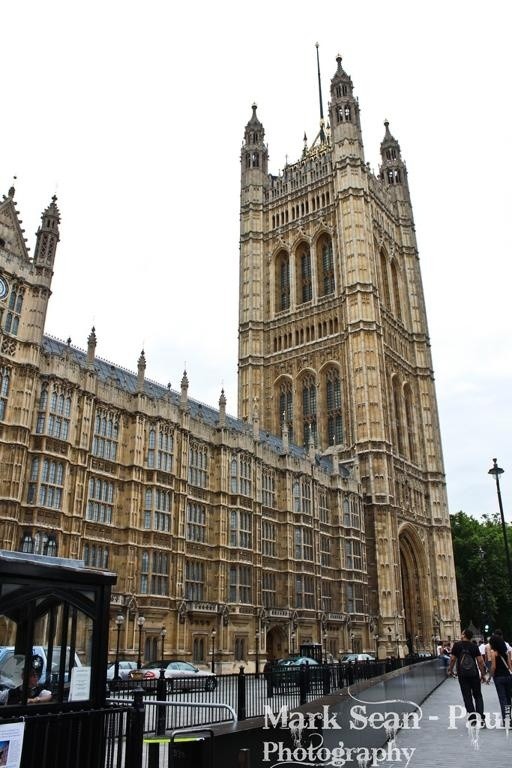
1, 665, 53, 706
449, 628, 488, 728
434, 629, 511, 681
485, 634, 512, 730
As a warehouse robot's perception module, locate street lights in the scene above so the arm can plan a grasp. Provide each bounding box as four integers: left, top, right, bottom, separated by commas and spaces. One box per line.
253, 629, 261, 678
475, 544, 488, 642
288, 629, 442, 661
488, 456, 512, 585
112, 606, 123, 691
159, 624, 167, 661
134, 610, 147, 669
208, 625, 219, 674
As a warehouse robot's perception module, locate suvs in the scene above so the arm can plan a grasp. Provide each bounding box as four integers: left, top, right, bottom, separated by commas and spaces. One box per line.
0, 645, 85, 694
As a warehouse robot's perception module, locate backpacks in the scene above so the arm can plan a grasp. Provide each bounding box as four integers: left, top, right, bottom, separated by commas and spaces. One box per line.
457, 641, 477, 679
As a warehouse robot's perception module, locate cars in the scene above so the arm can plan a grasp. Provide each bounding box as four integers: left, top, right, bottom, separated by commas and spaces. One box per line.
128, 660, 219, 693
263, 650, 436, 687
106, 661, 147, 691
